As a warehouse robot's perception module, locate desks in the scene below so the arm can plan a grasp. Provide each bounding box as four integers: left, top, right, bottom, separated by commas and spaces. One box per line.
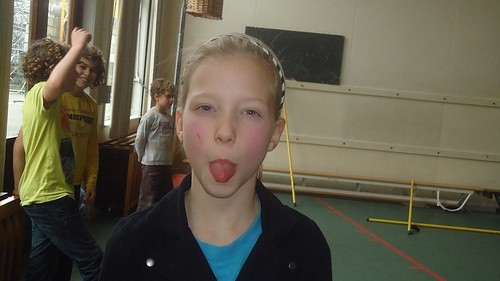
99, 132, 144, 219
0, 192, 33, 281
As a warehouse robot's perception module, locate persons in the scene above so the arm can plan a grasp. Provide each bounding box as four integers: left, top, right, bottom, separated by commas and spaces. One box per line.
134, 78, 176, 211
60, 46, 99, 212
11, 25, 100, 281
101, 32, 332, 281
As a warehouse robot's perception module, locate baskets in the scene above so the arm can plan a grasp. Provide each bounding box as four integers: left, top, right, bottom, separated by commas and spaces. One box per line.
186, 0, 224, 20
